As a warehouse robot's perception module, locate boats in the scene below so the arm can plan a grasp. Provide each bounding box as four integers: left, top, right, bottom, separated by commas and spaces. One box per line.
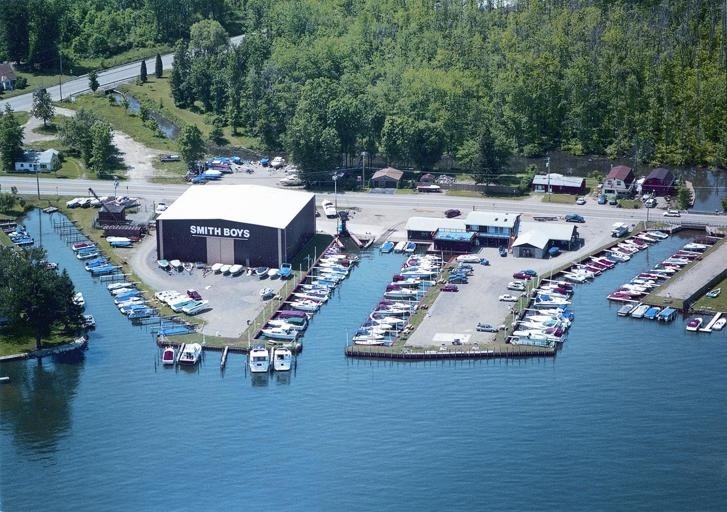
561, 256, 614, 282
651, 240, 706, 274
43, 207, 56, 213
155, 290, 207, 314
162, 346, 175, 366
77, 315, 95, 328
607, 231, 668, 263
607, 272, 670, 303
353, 255, 442, 345
0, 220, 34, 247
687, 318, 703, 331
664, 209, 681, 217
46, 261, 58, 271
53, 335, 89, 356
273, 347, 293, 370
706, 288, 723, 297
184, 264, 193, 272
289, 254, 357, 310
265, 311, 307, 341
158, 259, 169, 270
108, 283, 161, 323
71, 291, 85, 308
711, 316, 727, 330
249, 349, 269, 373
260, 287, 273, 296
531, 279, 574, 308
179, 343, 203, 365
150, 322, 195, 336
171, 259, 181, 271
72, 240, 121, 277
255, 263, 292, 278
617, 304, 677, 322
510, 309, 573, 347
211, 262, 243, 275
381, 241, 416, 253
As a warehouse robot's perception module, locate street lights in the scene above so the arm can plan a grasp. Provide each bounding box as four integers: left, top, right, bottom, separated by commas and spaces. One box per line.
332, 170, 344, 236
361, 152, 367, 187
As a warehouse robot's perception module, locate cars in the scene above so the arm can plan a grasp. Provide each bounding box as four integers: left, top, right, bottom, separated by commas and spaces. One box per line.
499, 294, 518, 302
449, 265, 473, 283
192, 156, 240, 182
160, 154, 181, 162
575, 198, 586, 205
565, 214, 585, 223
281, 165, 305, 185
441, 284, 457, 292
157, 202, 166, 211
643, 194, 651, 201
476, 323, 497, 332
513, 270, 536, 279
610, 200, 617, 204
322, 201, 336, 217
260, 157, 284, 167
457, 255, 481, 262
646, 198, 656, 207
508, 281, 525, 291
66, 196, 141, 207
596, 195, 607, 204
